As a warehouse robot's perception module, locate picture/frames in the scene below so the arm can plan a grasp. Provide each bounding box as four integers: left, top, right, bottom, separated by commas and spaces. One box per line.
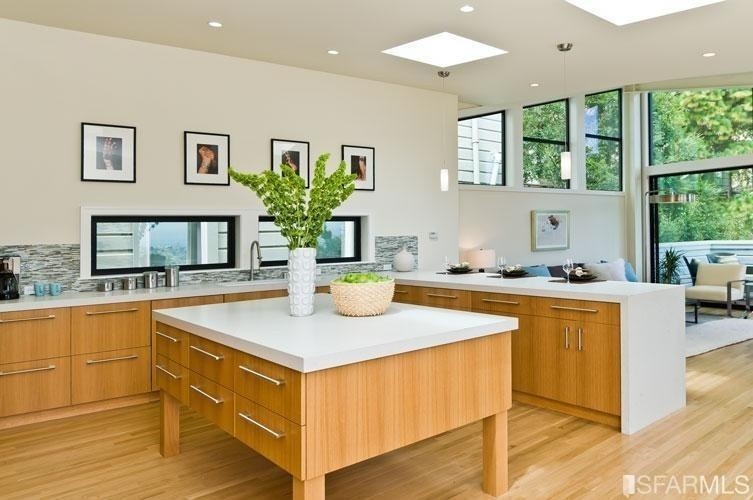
80, 122, 137, 184
339, 144, 375, 192
269, 139, 310, 190
182, 128, 231, 188
529, 208, 572, 252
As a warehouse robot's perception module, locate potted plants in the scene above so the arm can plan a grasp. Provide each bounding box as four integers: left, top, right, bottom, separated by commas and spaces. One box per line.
228, 152, 357, 318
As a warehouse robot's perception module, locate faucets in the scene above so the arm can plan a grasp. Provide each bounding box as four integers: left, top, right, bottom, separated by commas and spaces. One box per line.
247, 240, 264, 281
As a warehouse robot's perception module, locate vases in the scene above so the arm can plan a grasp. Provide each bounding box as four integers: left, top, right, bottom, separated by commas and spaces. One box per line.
392, 244, 416, 272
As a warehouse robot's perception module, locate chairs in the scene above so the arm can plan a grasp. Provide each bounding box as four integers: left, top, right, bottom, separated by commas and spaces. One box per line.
705, 252, 738, 264
682, 254, 698, 286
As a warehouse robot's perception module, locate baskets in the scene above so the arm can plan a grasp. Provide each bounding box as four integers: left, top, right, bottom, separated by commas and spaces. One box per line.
330, 276, 395, 317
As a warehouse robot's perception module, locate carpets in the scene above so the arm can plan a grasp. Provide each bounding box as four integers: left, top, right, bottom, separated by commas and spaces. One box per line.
671, 311, 751, 359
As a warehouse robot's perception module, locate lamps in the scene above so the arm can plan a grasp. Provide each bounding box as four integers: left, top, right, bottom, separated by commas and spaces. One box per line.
435, 70, 453, 194
558, 43, 573, 181
639, 184, 695, 282
461, 248, 496, 273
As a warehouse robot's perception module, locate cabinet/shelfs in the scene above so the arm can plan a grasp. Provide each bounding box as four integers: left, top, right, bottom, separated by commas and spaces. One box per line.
422, 289, 470, 311
0, 299, 72, 418
71, 298, 151, 408
151, 296, 224, 394
393, 283, 421, 304
528, 296, 620, 434
471, 293, 531, 406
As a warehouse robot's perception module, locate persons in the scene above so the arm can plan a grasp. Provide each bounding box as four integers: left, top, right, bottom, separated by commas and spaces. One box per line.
199, 147, 215, 175
100, 138, 119, 171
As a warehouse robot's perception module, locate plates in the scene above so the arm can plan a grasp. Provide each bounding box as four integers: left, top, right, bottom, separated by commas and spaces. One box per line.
562, 274, 597, 281
447, 268, 473, 273
497, 270, 527, 277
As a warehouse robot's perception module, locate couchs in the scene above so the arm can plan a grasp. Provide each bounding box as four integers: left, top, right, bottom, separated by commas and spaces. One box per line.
498, 261, 610, 282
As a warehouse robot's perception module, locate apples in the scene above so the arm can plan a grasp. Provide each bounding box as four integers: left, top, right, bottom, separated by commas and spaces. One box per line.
333, 272, 392, 285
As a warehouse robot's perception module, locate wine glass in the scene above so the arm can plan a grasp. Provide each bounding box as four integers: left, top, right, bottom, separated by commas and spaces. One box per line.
561, 256, 573, 285
496, 254, 506, 280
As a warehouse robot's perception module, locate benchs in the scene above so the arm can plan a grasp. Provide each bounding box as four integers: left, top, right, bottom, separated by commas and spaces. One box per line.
681, 262, 751, 321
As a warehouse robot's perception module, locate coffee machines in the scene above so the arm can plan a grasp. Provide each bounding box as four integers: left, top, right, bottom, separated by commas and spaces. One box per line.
0, 253, 21, 301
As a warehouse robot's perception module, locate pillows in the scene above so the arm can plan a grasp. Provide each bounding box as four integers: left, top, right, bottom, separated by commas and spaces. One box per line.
520, 264, 550, 277
622, 262, 639, 282
713, 254, 736, 263
691, 257, 698, 276
583, 259, 625, 282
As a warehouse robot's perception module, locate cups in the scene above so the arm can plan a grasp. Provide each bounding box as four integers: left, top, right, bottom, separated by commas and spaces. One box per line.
33, 283, 45, 296
48, 282, 61, 296
